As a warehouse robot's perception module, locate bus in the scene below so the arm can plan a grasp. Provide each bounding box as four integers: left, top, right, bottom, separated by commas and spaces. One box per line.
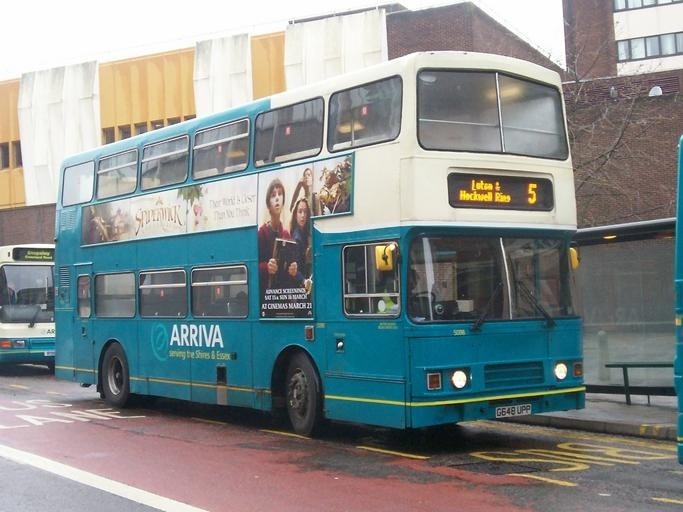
668, 131, 682, 465
1, 241, 56, 376
52, 49, 585, 441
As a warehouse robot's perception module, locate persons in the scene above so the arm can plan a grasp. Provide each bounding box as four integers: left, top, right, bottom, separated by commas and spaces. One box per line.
257, 168, 313, 318
